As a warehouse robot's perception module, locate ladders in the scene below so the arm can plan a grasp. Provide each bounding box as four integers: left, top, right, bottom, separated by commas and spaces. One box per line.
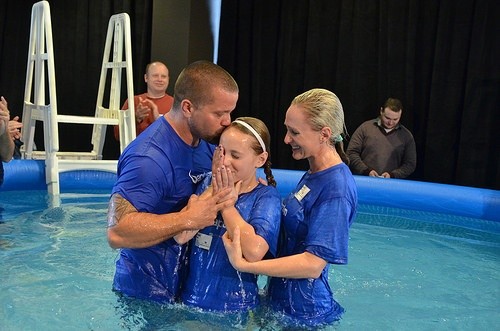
19, 1, 137, 208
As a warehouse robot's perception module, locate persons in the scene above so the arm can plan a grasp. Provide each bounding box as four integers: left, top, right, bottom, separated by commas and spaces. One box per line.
114, 62, 173, 142
346, 99, 416, 178
108, 61, 238, 303
0, 96, 23, 186
173, 117, 282, 313
221, 89, 358, 319
